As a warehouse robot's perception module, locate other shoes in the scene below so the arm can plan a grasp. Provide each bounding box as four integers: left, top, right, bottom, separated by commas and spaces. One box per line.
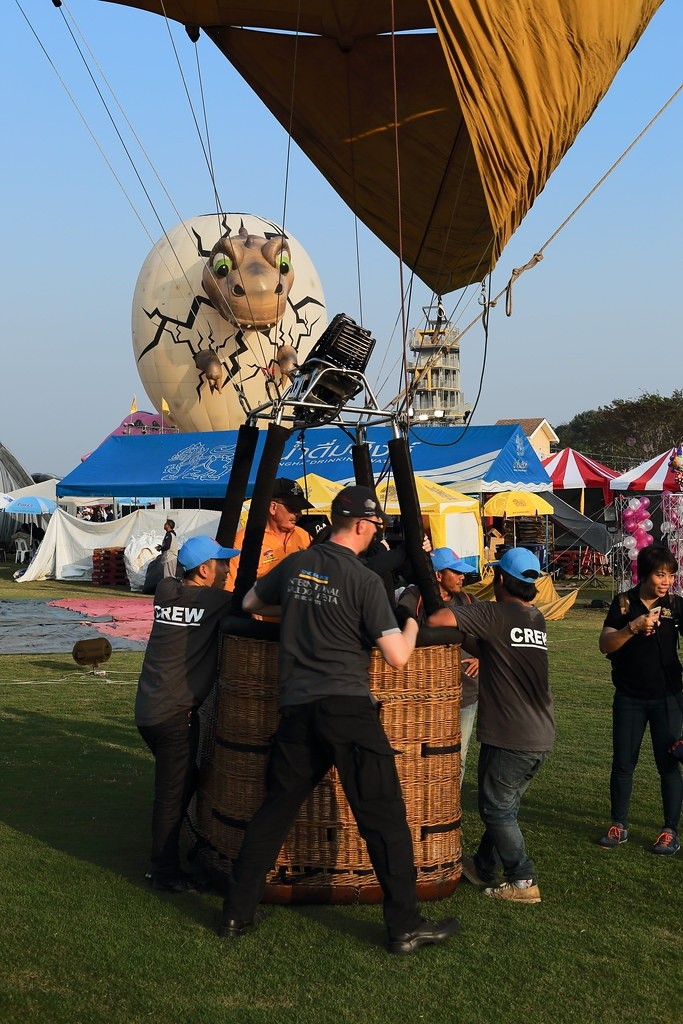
146, 867, 189, 892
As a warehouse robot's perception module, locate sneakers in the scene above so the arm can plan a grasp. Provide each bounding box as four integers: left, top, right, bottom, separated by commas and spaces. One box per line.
596, 820, 629, 850
388, 917, 460, 956
455, 851, 487, 885
218, 909, 267, 938
651, 828, 680, 857
482, 881, 542, 904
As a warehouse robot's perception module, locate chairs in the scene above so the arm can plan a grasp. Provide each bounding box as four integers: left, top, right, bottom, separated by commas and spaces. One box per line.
14, 538, 40, 564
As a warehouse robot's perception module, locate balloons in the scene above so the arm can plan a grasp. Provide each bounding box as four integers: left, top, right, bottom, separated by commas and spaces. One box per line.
622, 491, 683, 593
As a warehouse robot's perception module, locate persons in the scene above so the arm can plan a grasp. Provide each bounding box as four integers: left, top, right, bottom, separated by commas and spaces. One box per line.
426, 547, 556, 904
155, 520, 179, 578
79, 505, 115, 523
311, 509, 478, 784
223, 478, 316, 626
15, 523, 45, 550
598, 544, 683, 856
135, 535, 281, 891
218, 486, 460, 955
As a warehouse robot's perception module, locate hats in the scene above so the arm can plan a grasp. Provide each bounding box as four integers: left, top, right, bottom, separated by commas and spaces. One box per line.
331, 485, 389, 522
268, 477, 315, 510
484, 547, 541, 583
177, 534, 241, 571
428, 548, 477, 574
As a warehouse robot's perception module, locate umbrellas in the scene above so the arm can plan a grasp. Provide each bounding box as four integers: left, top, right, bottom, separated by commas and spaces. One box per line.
5, 496, 59, 557
481, 491, 554, 547
480, 491, 554, 548
0, 493, 16, 512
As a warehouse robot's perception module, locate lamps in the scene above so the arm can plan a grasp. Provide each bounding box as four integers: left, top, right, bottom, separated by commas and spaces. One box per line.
603, 507, 620, 534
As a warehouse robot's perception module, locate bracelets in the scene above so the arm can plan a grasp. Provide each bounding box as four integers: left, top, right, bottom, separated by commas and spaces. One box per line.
627, 622, 638, 635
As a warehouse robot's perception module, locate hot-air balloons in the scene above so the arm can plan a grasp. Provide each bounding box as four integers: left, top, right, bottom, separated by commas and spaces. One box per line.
13, 0, 682, 900
80, 412, 177, 461
0, 443, 72, 554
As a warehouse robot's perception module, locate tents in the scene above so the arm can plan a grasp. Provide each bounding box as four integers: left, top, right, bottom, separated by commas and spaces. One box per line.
540, 446, 682, 515
367, 477, 485, 575
6, 478, 113, 518
56, 423, 553, 581
236, 472, 347, 534
